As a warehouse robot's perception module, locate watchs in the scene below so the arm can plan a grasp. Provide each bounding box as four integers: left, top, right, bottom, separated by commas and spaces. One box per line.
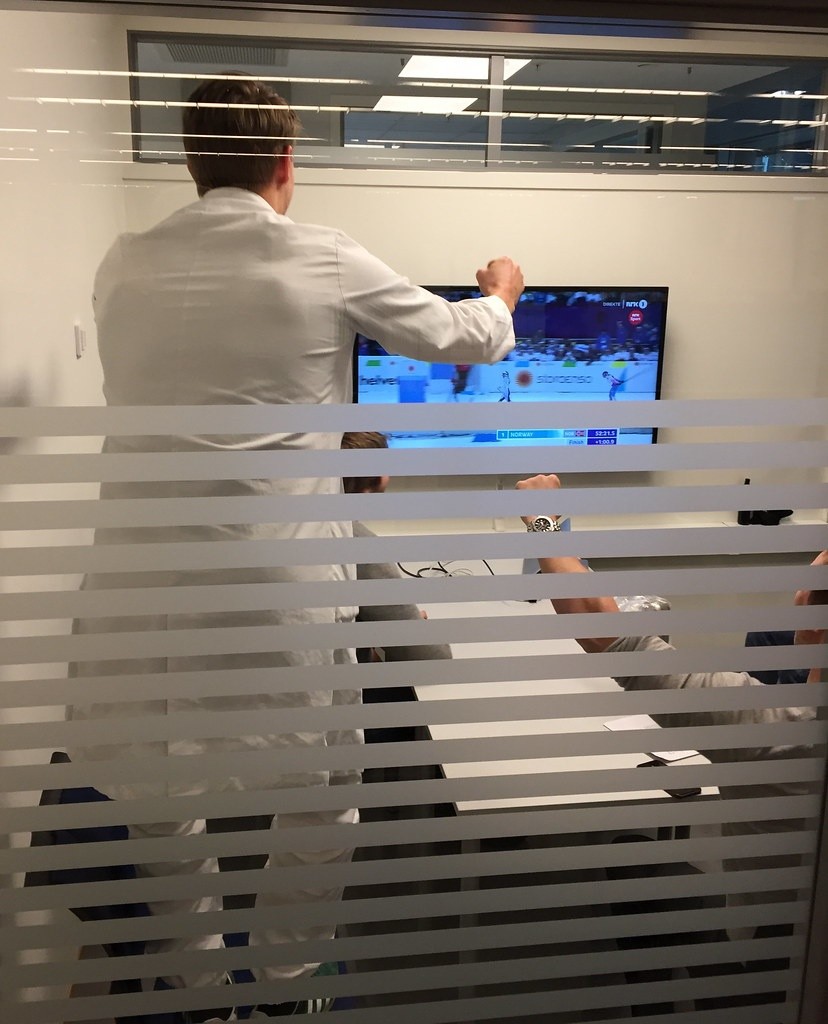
527, 516, 561, 532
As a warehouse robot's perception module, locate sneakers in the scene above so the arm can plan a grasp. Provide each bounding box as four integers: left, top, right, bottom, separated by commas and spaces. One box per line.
250, 960, 335, 1018
176, 971, 238, 1024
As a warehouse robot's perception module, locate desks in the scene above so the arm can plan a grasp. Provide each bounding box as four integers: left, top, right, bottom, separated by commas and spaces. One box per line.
411, 600, 722, 808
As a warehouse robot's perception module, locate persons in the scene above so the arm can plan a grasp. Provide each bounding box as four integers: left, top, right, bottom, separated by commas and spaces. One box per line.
452, 324, 659, 402
514, 473, 828, 969
63, 70, 523, 1024
341, 433, 452, 661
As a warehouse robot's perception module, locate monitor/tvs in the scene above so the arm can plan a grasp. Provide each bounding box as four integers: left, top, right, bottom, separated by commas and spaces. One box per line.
351, 285, 670, 450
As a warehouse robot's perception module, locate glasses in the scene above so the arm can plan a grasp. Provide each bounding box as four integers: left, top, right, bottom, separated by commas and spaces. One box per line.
394, 559, 496, 578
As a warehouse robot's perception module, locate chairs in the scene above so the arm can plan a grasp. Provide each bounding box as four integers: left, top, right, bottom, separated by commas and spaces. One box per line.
23, 752, 364, 1024
